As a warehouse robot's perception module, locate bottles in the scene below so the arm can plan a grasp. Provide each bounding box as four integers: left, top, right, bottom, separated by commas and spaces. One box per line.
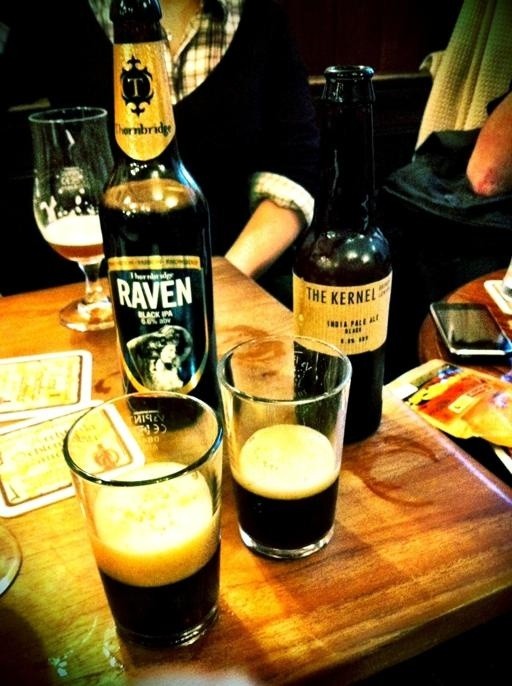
294, 62, 393, 442
99, 0, 220, 430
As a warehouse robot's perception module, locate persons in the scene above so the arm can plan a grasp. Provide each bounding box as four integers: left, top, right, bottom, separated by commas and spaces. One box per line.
465, 90, 512, 199
1, 0, 323, 311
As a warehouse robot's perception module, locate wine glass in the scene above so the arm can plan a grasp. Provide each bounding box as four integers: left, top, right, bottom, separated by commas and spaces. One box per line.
27, 105, 116, 333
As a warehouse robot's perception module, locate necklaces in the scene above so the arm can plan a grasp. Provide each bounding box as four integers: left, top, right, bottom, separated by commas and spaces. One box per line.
155, 17, 195, 52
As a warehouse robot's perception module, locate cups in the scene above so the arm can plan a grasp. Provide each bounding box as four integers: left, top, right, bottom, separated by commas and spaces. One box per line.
218, 331, 353, 561
64, 393, 225, 658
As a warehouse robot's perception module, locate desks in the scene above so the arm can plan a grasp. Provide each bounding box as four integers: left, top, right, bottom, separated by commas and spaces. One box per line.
416, 261, 512, 473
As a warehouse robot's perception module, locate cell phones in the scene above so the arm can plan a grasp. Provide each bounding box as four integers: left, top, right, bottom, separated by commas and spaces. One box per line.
430, 302, 512, 365
